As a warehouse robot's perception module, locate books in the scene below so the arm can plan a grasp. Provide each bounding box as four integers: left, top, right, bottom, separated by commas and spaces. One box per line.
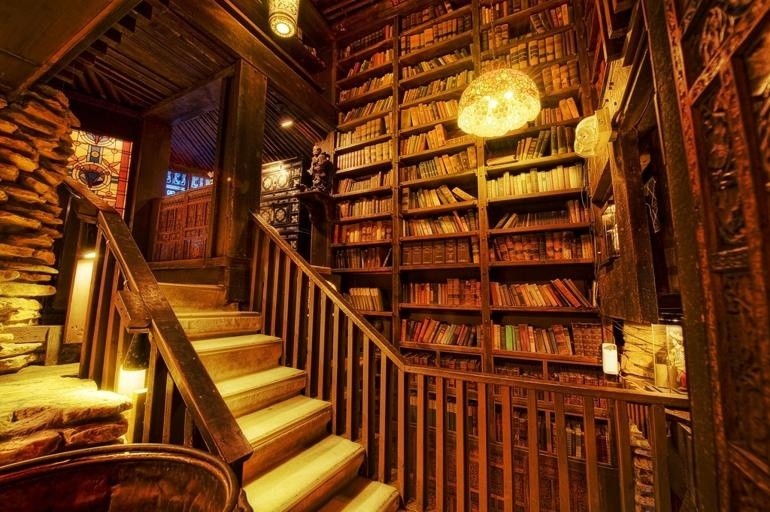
481, 1, 612, 462
335, 26, 393, 311
400, 0, 480, 438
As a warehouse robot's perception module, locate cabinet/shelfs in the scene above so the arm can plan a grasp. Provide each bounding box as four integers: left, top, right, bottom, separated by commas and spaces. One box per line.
320, 0, 634, 511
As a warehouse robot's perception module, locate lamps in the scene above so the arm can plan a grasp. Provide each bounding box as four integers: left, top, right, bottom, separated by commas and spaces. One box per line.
267, 0, 301, 39
452, 4, 544, 140
